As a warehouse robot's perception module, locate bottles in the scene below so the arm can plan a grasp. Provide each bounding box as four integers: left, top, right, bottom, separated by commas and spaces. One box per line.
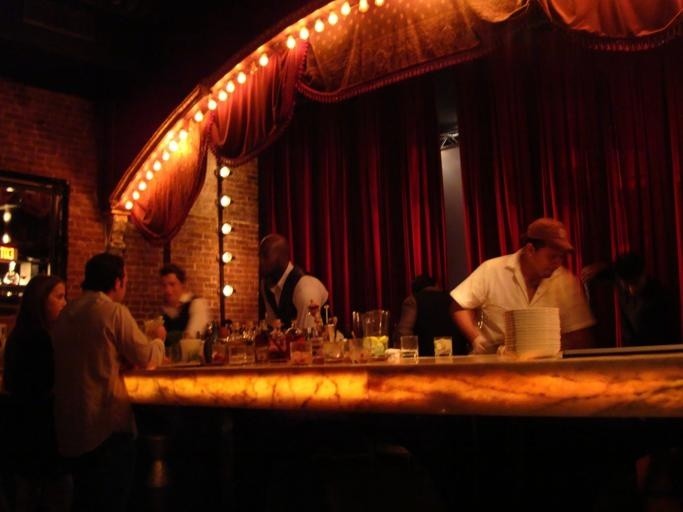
211, 304, 361, 365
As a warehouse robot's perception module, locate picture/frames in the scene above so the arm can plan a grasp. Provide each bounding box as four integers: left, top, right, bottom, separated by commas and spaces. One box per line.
0, 169, 71, 314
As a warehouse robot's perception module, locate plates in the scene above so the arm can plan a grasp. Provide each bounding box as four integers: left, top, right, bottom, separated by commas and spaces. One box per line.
504, 307, 563, 363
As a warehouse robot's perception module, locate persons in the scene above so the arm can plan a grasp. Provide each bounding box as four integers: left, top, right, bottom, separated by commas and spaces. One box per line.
151, 260, 211, 349
445, 215, 598, 357
397, 273, 456, 356
47, 253, 152, 471
255, 232, 329, 333
582, 250, 677, 347
0, 273, 68, 394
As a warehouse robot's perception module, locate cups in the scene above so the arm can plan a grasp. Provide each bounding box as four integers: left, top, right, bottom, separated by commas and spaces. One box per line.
401, 336, 418, 364
144, 316, 164, 336
434, 336, 453, 356
180, 338, 206, 365
361, 309, 389, 358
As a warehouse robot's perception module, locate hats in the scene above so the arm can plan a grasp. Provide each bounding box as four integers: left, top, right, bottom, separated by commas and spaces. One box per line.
526, 216, 574, 253
